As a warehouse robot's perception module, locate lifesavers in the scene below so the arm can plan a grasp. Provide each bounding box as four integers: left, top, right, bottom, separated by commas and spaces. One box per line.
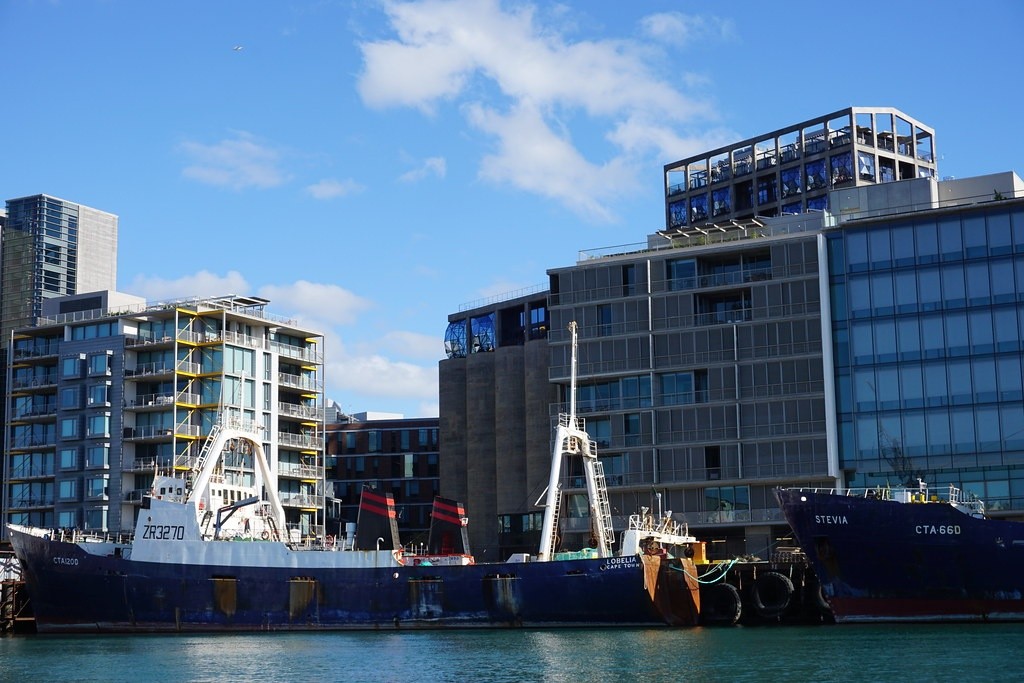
325, 535, 333, 546
749, 571, 796, 619
815, 585, 831, 609
699, 582, 745, 626
261, 531, 269, 540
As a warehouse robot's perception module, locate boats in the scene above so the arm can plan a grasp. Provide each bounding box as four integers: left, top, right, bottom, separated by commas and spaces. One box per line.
4, 320, 768, 630
776, 483, 1024, 626
